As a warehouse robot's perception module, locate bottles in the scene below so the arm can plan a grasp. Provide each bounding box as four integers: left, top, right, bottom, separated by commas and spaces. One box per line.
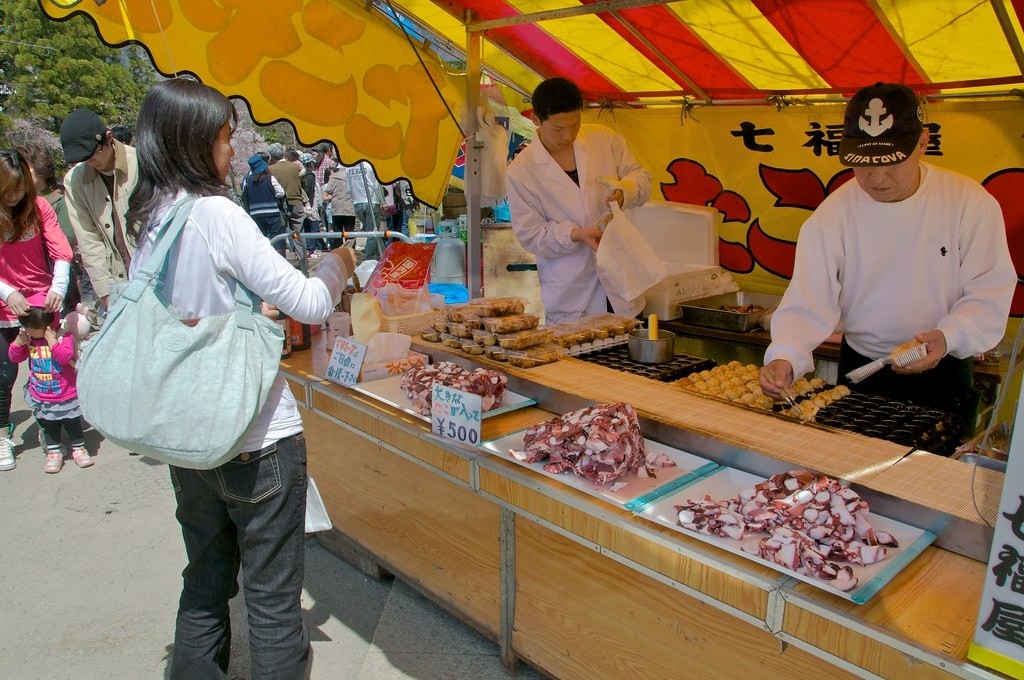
1000, 352, 1013, 376
292, 319, 311, 352
276, 311, 292, 360
486, 214, 491, 224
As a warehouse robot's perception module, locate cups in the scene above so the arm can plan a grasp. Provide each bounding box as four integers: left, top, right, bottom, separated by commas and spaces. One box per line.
326, 312, 353, 350
345, 288, 363, 312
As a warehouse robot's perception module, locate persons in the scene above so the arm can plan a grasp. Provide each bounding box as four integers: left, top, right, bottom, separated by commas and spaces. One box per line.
124, 78, 356, 680
506, 78, 652, 325
759, 83, 1019, 438
0, 109, 411, 472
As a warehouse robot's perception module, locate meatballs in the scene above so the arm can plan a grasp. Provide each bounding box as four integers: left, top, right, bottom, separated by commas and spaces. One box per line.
665, 360, 853, 420
419, 298, 633, 368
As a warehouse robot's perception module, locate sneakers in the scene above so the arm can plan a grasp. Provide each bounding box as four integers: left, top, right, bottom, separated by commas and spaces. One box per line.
0, 423, 16, 471
45, 453, 63, 473
35, 417, 49, 455
73, 449, 94, 468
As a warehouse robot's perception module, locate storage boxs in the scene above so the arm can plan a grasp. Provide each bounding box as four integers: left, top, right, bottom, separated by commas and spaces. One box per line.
624, 198, 724, 321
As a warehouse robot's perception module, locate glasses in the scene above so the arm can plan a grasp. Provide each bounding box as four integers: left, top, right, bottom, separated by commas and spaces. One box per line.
95, 143, 104, 160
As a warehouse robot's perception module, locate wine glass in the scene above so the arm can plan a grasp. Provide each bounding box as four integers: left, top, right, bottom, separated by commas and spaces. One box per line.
416, 219, 425, 236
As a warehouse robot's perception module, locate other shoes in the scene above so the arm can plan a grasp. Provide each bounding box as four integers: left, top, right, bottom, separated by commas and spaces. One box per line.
323, 247, 331, 252
305, 202, 311, 210
289, 253, 298, 261
307, 252, 322, 260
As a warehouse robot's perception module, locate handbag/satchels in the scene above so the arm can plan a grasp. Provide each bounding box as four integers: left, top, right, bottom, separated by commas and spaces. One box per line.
387, 204, 396, 215
77, 197, 285, 469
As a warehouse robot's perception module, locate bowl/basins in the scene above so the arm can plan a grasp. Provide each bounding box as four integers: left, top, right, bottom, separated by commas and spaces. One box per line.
628, 329, 675, 363
415, 234, 437, 243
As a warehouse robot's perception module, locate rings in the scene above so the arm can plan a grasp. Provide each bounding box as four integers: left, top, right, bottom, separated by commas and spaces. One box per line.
55, 302, 58, 305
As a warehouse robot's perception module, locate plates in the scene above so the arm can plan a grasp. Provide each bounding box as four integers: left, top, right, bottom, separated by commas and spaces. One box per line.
631, 465, 947, 604
476, 422, 719, 511
350, 372, 537, 423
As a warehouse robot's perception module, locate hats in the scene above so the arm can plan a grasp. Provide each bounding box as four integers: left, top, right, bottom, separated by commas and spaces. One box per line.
248, 155, 268, 174
838, 82, 924, 167
60, 109, 106, 163
300, 153, 317, 163
255, 152, 269, 162
